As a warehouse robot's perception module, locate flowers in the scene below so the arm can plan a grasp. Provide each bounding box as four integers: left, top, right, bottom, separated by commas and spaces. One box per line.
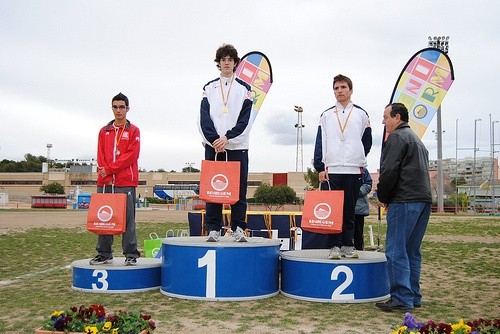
392, 312, 500, 334
42, 303, 156, 334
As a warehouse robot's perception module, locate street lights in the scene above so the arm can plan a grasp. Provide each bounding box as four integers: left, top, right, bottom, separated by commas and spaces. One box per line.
474, 119, 482, 216
428, 35, 449, 213
294, 105, 304, 173
186, 162, 195, 173
492, 120, 499, 214
46, 143, 52, 159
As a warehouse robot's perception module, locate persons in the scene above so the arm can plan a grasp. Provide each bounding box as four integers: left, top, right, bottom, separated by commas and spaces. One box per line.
313, 74, 372, 258
376, 102, 432, 308
197, 44, 253, 241
352, 166, 372, 251
89, 91, 140, 266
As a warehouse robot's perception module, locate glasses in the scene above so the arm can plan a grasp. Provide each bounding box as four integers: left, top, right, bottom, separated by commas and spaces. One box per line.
111, 105, 127, 110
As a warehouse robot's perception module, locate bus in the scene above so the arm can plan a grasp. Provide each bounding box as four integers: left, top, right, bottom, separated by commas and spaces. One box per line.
154, 184, 199, 203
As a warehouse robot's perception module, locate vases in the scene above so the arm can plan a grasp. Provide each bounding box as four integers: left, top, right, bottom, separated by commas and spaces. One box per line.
35, 325, 150, 334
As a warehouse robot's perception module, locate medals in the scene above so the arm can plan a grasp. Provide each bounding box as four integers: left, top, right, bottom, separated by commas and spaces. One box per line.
340, 134, 345, 141
221, 106, 228, 113
115, 149, 120, 155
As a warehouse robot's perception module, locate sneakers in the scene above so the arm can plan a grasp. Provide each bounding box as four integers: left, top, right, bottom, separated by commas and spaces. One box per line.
90, 255, 113, 265
125, 257, 136, 265
376, 299, 413, 311
414, 305, 421, 307
341, 246, 359, 257
206, 230, 220, 242
234, 226, 247, 242
328, 246, 341, 258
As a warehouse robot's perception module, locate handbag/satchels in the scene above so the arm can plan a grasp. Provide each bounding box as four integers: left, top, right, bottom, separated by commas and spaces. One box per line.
301, 179, 344, 234
87, 184, 127, 235
199, 150, 240, 205
